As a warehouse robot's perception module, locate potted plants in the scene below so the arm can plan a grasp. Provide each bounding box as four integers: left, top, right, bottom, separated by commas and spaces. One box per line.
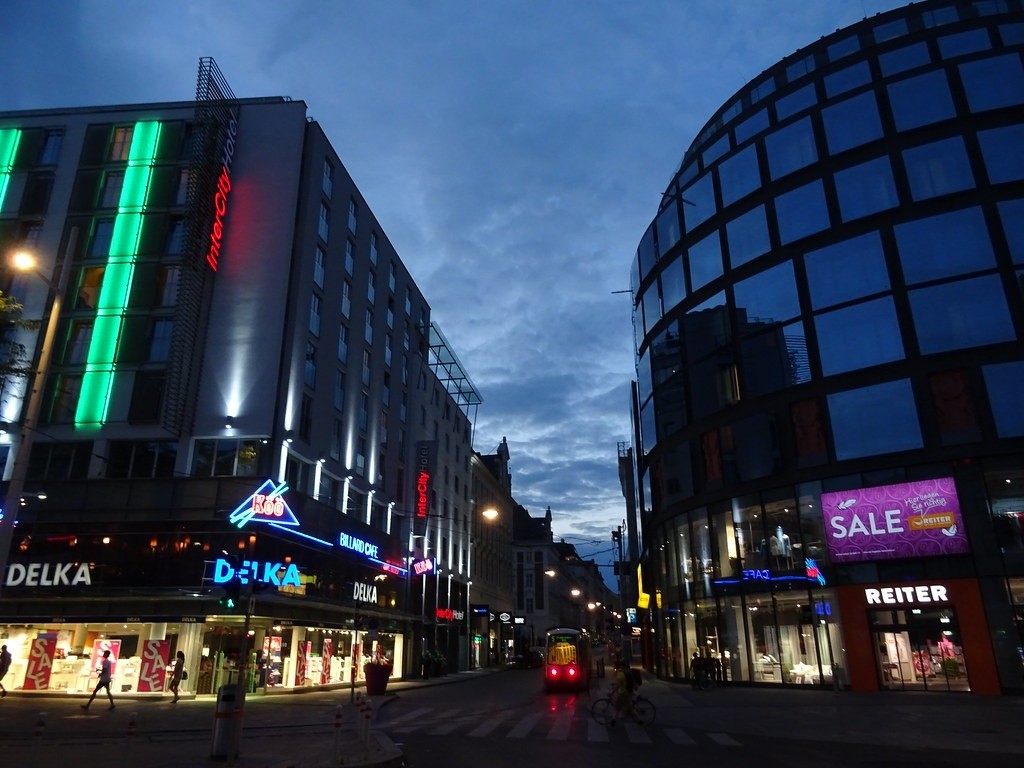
433, 650, 447, 677
422, 650, 434, 680
364, 661, 393, 695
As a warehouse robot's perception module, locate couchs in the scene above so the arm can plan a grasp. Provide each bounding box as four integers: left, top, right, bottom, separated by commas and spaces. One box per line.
790, 664, 833, 684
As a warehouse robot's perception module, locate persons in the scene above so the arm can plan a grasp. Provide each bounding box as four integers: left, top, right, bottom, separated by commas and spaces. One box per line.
610, 660, 643, 726
0, 645, 11, 698
689, 652, 722, 692
168, 652, 184, 703
80, 651, 116, 712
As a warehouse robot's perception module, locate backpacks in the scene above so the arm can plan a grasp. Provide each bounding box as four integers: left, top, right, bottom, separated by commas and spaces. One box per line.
629, 668, 642, 686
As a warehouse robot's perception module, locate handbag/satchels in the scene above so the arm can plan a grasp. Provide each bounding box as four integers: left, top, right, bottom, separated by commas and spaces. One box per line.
182, 671, 187, 679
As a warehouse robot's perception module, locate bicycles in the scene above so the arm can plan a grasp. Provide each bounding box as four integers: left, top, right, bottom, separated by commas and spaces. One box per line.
590, 685, 657, 727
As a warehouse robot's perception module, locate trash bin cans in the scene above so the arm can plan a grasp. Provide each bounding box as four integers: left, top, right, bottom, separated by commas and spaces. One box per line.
209, 682, 246, 762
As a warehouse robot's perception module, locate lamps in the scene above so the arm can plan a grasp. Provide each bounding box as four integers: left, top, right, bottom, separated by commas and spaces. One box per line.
391, 497, 396, 505
347, 469, 354, 479
320, 453, 326, 463
225, 416, 234, 429
286, 430, 294, 443
370, 484, 376, 494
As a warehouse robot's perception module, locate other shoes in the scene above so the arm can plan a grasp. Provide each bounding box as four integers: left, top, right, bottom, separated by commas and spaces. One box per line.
80, 705, 89, 709
1, 690, 7, 697
610, 719, 616, 727
171, 696, 179, 703
108, 705, 115, 710
637, 719, 645, 725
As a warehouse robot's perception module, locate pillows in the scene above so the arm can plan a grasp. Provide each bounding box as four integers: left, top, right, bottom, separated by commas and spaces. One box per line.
795, 662, 807, 672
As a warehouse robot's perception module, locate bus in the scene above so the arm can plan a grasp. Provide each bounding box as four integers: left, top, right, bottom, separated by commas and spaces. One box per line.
545, 628, 591, 689
